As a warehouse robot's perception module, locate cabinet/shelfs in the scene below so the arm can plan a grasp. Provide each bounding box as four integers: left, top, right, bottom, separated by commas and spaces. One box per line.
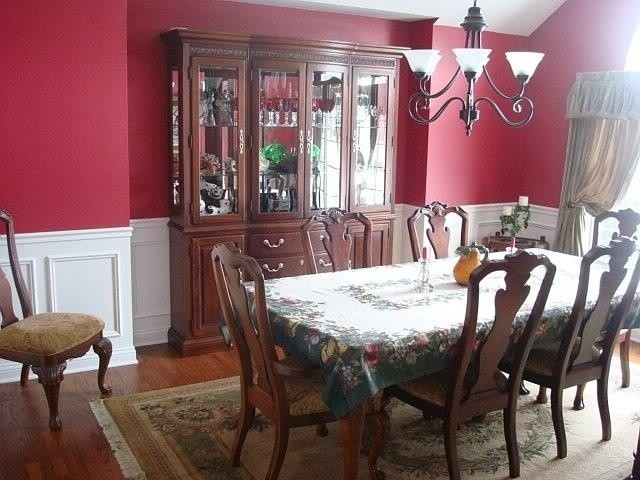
154, 25, 414, 356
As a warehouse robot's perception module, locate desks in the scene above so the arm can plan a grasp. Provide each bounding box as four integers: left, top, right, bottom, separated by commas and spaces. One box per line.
223, 242, 638, 469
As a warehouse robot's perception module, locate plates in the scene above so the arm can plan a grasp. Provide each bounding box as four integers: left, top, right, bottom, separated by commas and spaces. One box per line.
261, 142, 290, 169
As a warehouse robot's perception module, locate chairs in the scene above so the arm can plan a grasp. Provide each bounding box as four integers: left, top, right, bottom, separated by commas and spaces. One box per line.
210, 239, 392, 478
471, 233, 639, 458
0, 210, 112, 431
368, 248, 559, 474
539, 207, 638, 412
402, 198, 472, 262
297, 207, 375, 272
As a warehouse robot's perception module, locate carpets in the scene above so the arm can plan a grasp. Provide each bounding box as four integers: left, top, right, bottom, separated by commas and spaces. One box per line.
87, 345, 638, 478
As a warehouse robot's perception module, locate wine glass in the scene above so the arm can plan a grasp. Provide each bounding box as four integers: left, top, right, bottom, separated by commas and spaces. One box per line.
370, 105, 385, 129
311, 98, 335, 129
265, 97, 300, 127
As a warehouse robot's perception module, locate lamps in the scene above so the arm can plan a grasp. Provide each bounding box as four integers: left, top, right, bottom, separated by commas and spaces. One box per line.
401, 0, 545, 137
